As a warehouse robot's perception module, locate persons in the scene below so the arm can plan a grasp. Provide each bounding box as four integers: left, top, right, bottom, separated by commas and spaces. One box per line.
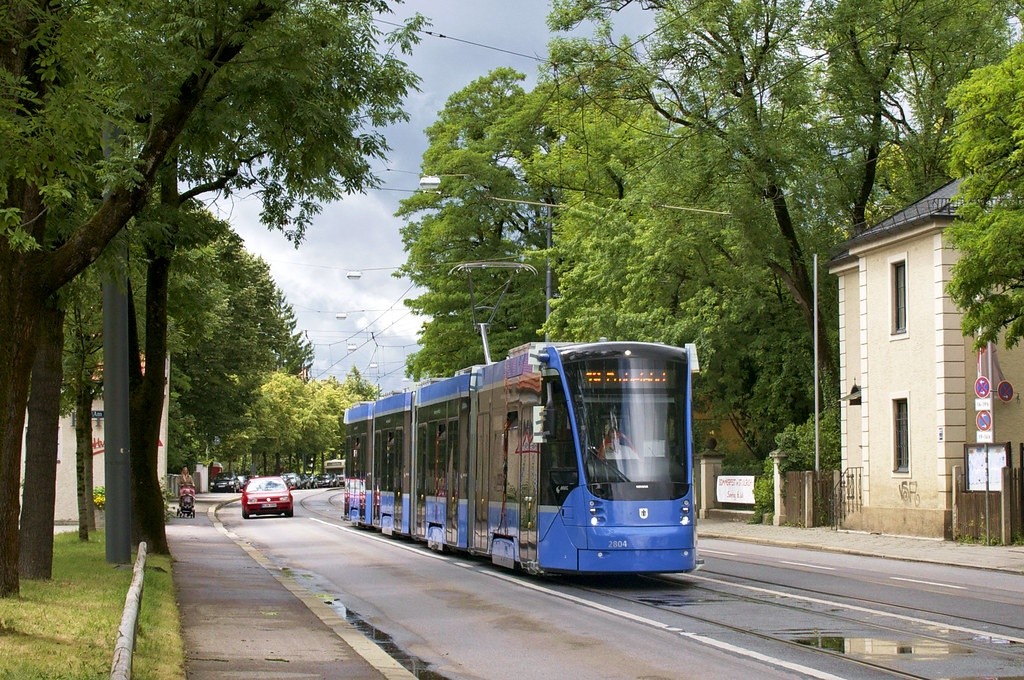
182, 489, 197, 508
596, 427, 639, 459
177, 467, 195, 488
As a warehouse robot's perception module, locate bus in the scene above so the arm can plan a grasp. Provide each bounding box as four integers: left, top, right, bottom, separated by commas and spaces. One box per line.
324, 459, 345, 484
343, 264, 705, 575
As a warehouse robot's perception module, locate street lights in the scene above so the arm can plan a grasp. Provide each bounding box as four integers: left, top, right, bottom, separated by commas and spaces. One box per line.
419, 172, 553, 342
419, 171, 561, 342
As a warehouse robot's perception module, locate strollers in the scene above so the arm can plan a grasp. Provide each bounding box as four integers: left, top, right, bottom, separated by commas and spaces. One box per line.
177, 486, 195, 518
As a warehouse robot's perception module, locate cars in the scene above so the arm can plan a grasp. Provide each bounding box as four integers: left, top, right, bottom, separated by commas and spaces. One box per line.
239, 476, 295, 519
280, 472, 339, 490
210, 473, 246, 493
